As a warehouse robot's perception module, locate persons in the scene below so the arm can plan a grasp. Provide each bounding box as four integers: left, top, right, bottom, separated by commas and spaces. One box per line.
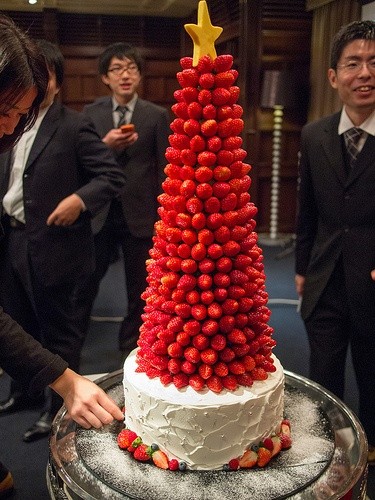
0, 15, 170, 492
294, 20, 375, 459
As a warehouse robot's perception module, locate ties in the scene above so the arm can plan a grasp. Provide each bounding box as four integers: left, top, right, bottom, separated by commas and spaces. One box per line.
115, 106, 128, 127
346, 127, 364, 169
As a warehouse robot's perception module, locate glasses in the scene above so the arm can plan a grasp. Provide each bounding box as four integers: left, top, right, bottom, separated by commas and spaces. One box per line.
109, 66, 139, 75
338, 59, 375, 72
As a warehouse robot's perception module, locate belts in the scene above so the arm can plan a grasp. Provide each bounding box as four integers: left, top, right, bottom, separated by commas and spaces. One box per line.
7, 214, 27, 229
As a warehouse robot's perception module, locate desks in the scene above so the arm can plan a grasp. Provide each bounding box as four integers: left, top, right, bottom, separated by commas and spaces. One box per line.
47, 369, 368, 500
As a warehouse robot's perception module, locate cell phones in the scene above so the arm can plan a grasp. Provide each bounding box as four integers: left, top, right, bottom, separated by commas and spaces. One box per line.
122, 124, 135, 135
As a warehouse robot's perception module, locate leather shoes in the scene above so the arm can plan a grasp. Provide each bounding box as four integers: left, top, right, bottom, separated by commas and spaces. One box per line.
22, 410, 54, 444
0, 395, 19, 416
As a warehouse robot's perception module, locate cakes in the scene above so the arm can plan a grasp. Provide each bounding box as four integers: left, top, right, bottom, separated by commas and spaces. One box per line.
118, 1, 292, 468
